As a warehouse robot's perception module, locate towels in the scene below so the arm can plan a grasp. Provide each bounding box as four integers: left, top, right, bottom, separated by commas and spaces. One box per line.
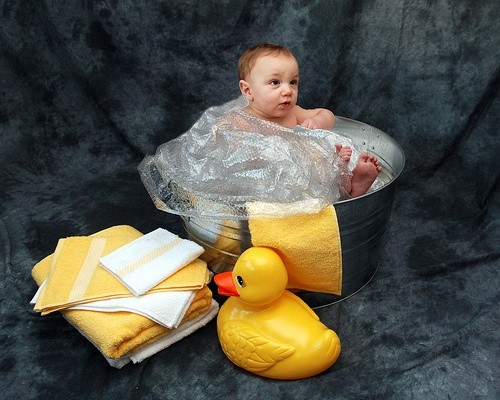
29, 223, 219, 371
246, 199, 345, 298
99, 227, 205, 297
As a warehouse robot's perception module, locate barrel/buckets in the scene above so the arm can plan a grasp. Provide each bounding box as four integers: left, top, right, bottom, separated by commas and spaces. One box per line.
157, 116, 405, 309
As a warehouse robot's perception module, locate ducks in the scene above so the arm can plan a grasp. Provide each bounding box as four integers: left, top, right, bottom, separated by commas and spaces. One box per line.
214, 247, 341, 381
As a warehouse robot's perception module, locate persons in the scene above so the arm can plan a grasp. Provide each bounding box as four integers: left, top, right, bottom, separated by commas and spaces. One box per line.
214, 44, 382, 198
